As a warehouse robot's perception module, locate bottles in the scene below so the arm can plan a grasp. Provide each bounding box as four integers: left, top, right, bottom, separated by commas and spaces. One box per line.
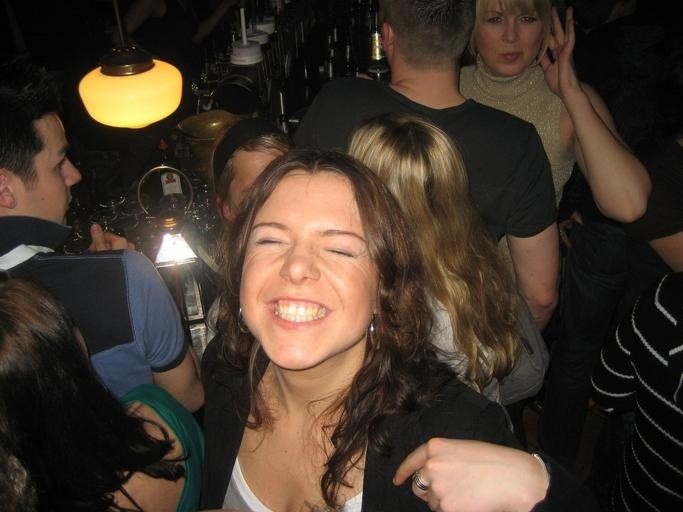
326, 0, 380, 85
159, 138, 187, 204
228, 0, 305, 111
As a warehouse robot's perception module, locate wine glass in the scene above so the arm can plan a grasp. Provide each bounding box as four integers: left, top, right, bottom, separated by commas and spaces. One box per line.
63, 155, 150, 256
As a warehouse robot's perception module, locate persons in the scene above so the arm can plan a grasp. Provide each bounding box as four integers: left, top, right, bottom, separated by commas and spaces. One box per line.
3, 1, 682, 511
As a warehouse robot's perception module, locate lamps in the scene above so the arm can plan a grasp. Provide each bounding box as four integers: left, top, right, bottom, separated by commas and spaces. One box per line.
230, 1, 278, 66
150, 230, 198, 307
75, 0, 185, 134
